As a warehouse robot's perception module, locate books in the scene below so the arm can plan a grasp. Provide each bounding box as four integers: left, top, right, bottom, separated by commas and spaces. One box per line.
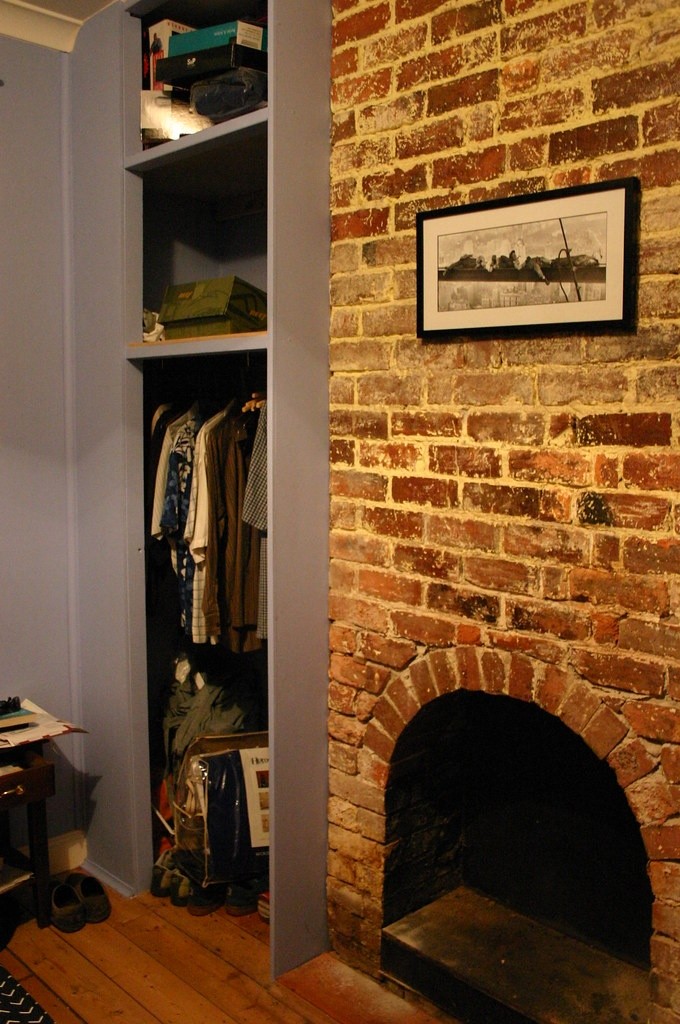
0, 708, 36, 728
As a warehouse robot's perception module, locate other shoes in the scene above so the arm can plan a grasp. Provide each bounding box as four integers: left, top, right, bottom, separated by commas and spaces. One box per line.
17, 875, 86, 933
58, 872, 111, 923
151, 846, 179, 896
170, 870, 199, 907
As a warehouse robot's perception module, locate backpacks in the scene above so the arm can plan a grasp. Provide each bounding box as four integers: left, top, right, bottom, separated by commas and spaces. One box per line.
164, 655, 245, 788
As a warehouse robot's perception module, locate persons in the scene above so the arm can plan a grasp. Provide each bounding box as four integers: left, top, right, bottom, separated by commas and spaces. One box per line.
150, 33, 162, 53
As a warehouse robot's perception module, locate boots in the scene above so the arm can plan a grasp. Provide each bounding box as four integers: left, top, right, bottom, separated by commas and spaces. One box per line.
186, 753, 227, 916
224, 750, 269, 915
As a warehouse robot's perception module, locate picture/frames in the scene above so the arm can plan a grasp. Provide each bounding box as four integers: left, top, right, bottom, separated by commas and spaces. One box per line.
415, 174, 641, 339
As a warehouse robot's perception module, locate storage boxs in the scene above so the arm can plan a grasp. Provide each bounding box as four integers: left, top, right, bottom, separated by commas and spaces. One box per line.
145, 20, 265, 93
156, 276, 267, 340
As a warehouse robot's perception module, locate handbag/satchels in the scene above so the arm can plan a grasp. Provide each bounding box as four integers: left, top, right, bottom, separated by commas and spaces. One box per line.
172, 730, 269, 859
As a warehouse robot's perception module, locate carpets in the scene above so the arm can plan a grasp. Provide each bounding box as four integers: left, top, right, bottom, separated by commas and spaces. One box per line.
0, 964, 56, 1024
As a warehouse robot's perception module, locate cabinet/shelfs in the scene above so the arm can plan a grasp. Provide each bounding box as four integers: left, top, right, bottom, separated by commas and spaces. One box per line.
122, 0, 330, 979
0, 732, 58, 928
